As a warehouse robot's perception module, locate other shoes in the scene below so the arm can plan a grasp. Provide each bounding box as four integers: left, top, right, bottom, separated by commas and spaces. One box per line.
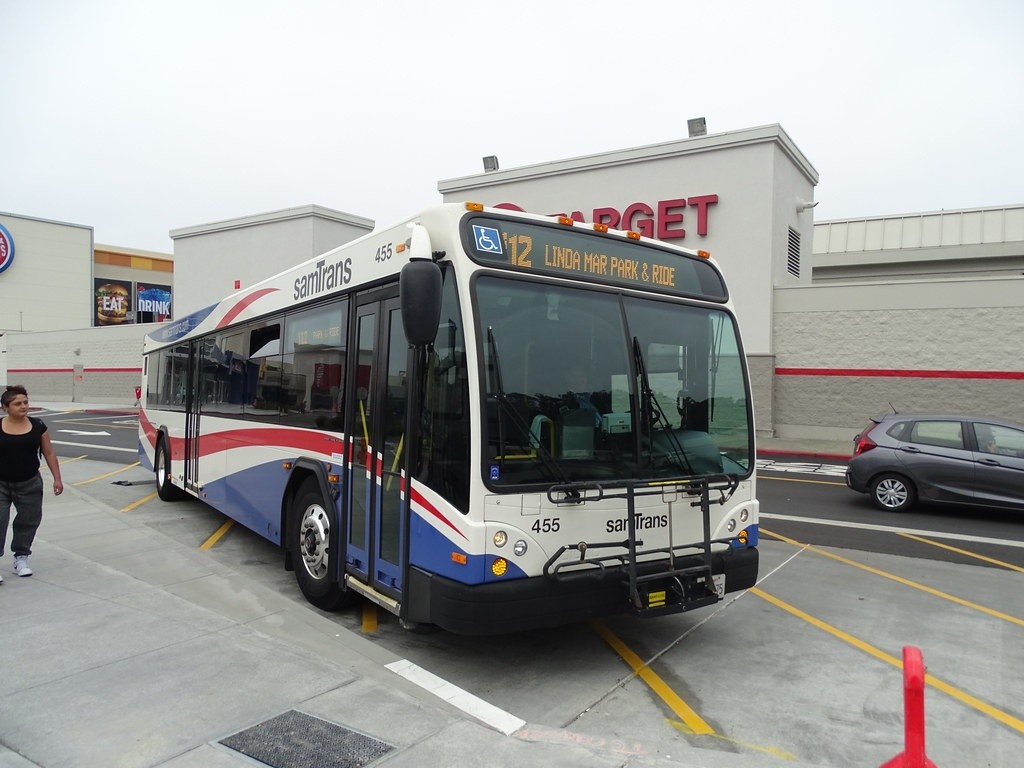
12, 555, 33, 577
0, 576, 3, 582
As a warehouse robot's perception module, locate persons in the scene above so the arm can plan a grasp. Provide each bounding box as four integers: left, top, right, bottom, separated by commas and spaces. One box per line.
0, 384, 64, 585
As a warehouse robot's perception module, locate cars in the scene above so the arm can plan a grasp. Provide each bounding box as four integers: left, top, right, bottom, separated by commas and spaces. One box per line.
844, 402, 1024, 517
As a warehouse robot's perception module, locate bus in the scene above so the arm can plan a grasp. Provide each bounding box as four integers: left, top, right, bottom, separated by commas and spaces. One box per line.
137, 200, 760, 637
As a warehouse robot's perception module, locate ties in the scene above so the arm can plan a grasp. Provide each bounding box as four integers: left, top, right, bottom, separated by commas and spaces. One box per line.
566, 391, 580, 410
